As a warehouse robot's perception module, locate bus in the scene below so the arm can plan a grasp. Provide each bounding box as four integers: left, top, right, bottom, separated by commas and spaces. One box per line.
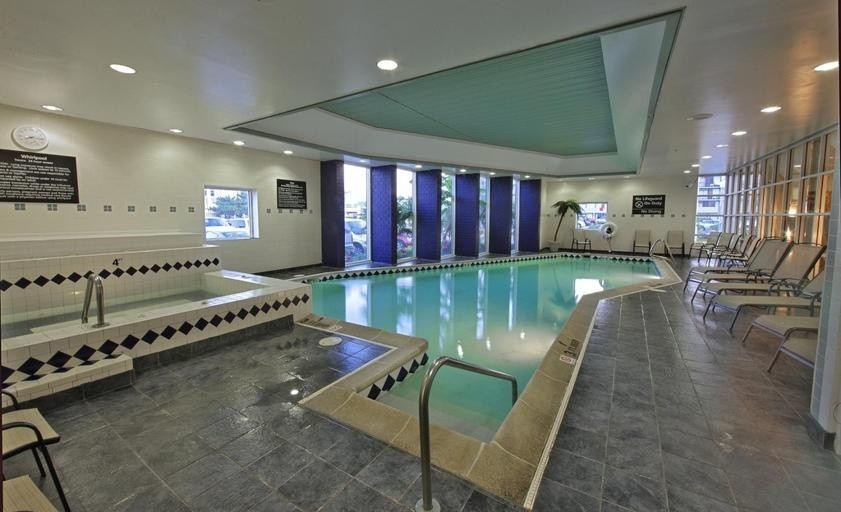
697, 220, 720, 232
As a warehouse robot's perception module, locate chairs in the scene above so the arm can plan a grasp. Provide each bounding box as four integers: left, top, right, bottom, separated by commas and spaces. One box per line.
632, 229, 685, 259
571, 229, 592, 253
1, 388, 70, 512
683, 231, 827, 373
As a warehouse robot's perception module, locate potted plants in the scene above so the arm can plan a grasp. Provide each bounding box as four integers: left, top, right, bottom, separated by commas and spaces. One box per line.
546, 198, 585, 252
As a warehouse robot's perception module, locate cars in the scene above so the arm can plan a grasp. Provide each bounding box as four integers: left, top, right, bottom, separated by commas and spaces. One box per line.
345, 217, 367, 256
577, 211, 607, 228
205, 217, 250, 238
397, 233, 411, 249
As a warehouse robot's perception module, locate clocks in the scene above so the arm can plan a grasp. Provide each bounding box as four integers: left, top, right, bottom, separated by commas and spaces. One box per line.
13, 124, 50, 151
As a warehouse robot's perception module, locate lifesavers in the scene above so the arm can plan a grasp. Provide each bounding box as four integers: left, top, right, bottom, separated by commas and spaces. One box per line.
601, 223, 617, 239
